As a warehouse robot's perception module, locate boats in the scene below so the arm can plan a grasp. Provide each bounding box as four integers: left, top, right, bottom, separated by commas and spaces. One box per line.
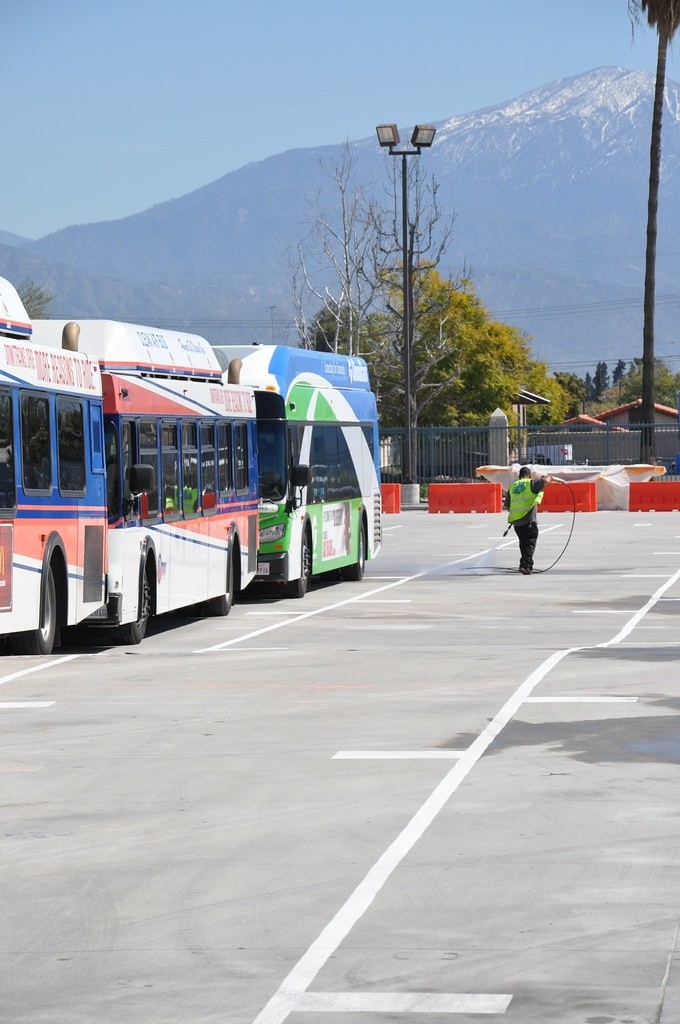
475, 462, 667, 511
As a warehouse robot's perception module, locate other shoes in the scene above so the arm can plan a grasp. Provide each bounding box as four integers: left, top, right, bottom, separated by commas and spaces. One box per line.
520, 568, 530, 575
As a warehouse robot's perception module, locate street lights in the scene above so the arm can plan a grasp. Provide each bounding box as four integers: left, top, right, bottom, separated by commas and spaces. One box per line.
375, 123, 437, 483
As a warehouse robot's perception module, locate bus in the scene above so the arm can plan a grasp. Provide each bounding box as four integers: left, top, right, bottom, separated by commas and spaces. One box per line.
0, 272, 109, 655
29, 317, 259, 647
211, 342, 383, 597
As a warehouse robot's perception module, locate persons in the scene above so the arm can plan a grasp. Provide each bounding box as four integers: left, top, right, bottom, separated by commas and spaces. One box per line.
505, 467, 551, 575
164, 478, 197, 513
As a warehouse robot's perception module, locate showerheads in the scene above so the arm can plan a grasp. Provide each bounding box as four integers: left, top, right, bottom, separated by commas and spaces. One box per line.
502, 523, 513, 537
